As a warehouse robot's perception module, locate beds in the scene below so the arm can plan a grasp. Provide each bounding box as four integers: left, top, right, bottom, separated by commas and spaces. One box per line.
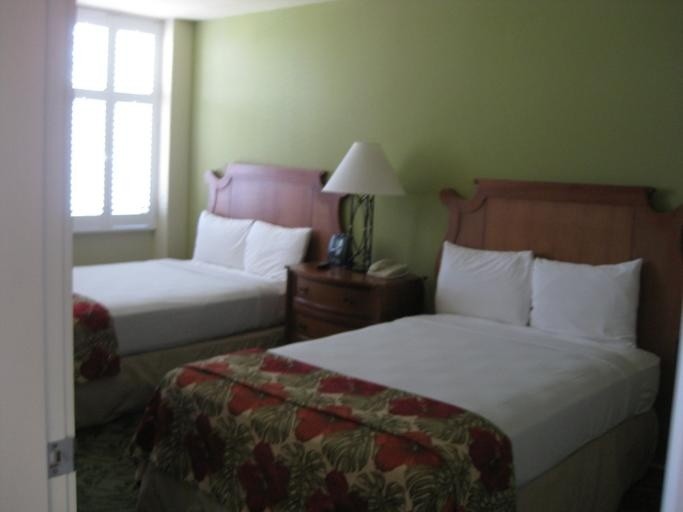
123, 172, 681, 511
70, 158, 348, 430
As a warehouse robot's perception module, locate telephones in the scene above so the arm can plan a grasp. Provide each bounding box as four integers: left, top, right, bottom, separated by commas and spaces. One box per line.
368, 258, 409, 280
327, 233, 350, 266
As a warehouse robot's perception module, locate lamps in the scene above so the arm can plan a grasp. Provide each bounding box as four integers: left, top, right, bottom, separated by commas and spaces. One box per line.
319, 139, 407, 275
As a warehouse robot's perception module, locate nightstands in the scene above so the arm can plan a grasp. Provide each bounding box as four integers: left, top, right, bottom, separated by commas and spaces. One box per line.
280, 258, 429, 344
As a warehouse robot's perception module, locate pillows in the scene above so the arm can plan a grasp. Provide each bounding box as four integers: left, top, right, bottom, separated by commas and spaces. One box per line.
527, 256, 648, 350
431, 237, 534, 329
191, 208, 255, 273
243, 219, 313, 284
